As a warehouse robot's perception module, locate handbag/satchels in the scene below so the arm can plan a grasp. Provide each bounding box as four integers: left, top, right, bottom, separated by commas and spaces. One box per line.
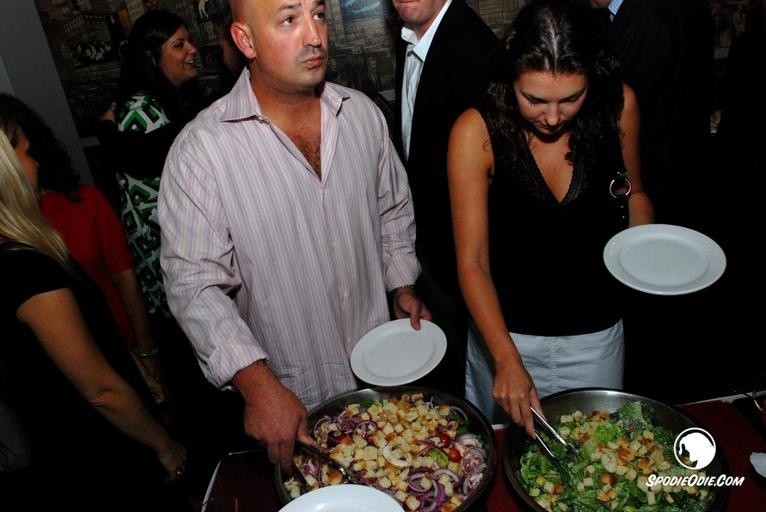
133, 345, 162, 359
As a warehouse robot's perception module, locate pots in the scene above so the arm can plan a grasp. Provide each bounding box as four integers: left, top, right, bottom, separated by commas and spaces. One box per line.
501, 387, 732, 512
277, 386, 498, 512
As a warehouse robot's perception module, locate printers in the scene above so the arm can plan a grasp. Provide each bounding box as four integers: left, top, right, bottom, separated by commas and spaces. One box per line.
530, 405, 584, 475
290, 440, 375, 494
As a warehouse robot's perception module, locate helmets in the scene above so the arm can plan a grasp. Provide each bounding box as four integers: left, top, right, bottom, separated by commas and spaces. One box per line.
278, 483, 405, 512
349, 317, 447, 387
602, 223, 727, 297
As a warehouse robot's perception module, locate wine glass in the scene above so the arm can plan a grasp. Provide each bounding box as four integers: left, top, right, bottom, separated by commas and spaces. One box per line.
176, 468, 185, 480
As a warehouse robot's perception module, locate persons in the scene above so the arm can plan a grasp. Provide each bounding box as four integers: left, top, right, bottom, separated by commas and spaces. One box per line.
105, 9, 204, 392
153, 0, 437, 478
445, 2, 655, 441
581, 0, 715, 227
0, 114, 191, 511
93, 1, 245, 183
366, 1, 510, 400
0, 91, 180, 442
